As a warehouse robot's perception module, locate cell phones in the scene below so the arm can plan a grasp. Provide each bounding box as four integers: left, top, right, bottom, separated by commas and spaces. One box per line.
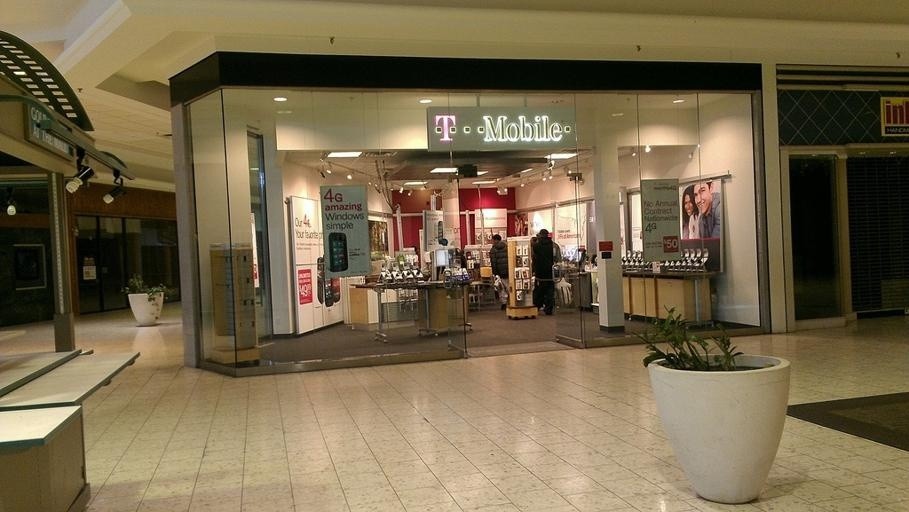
329, 232, 348, 272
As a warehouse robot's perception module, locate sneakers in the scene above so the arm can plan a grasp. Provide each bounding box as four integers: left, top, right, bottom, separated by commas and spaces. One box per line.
500, 303, 506, 310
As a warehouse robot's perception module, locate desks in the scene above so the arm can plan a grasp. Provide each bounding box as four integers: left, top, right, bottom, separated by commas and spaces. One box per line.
352, 281, 441, 345
416, 282, 474, 338
620, 267, 720, 330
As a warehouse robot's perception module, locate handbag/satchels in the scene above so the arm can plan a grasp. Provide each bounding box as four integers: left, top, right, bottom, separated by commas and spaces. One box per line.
493, 278, 509, 304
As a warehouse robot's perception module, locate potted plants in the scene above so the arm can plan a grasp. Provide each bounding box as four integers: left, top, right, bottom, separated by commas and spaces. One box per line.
622, 302, 797, 506
118, 273, 177, 326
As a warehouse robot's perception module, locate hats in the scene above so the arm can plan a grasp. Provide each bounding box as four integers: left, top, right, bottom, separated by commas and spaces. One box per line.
536, 228, 552, 240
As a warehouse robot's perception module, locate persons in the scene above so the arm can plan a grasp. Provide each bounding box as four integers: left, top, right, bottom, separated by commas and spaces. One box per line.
531, 237, 538, 254
532, 230, 562, 315
693, 181, 721, 238
465, 252, 476, 265
681, 184, 700, 239
489, 234, 510, 310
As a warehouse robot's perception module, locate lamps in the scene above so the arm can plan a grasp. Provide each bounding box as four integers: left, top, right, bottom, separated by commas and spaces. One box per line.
497, 163, 576, 196
325, 162, 413, 198
